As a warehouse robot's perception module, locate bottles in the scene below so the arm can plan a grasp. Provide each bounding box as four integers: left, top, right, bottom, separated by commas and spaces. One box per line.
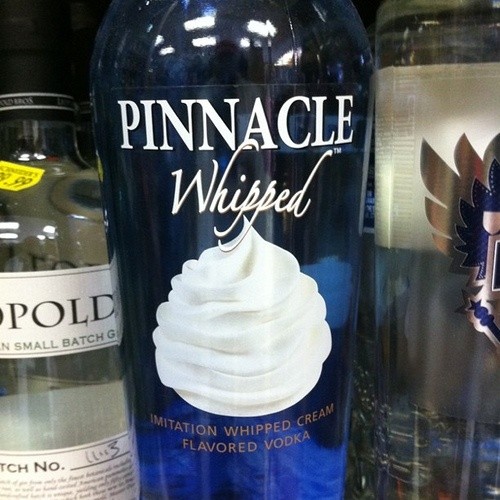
1, 1, 142, 500
93, 2, 375, 499
354, 1, 500, 499
